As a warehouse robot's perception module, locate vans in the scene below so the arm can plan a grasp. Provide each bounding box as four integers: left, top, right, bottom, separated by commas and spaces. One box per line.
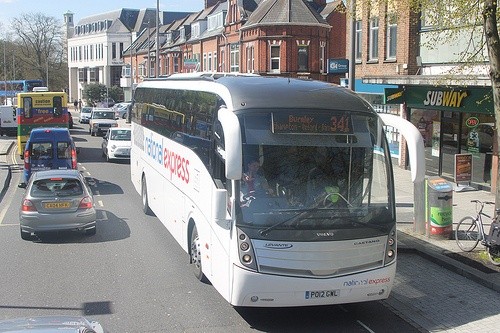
20, 126, 80, 191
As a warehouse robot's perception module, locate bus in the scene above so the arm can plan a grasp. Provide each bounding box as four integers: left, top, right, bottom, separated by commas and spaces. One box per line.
16, 87, 70, 156
130, 72, 425, 308
0, 80, 43, 137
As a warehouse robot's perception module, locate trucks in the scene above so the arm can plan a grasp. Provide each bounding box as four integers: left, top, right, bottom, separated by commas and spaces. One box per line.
88, 107, 119, 137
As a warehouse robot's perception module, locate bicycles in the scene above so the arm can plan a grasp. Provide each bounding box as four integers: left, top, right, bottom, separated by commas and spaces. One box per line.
455, 199, 500, 266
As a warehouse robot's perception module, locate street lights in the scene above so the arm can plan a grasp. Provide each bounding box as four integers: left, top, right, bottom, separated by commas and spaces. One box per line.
143, 22, 151, 77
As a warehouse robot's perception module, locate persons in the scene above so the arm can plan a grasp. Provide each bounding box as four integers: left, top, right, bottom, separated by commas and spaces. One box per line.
78, 98, 82, 112
74, 98, 78, 111
93, 100, 107, 108
239, 158, 268, 196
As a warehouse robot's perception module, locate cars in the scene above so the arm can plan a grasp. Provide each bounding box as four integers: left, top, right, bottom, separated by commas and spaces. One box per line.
101, 127, 131, 162
18, 170, 97, 240
78, 103, 132, 124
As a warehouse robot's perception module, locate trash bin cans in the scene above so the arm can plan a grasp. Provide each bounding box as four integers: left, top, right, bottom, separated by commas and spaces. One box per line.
424, 176, 453, 239
